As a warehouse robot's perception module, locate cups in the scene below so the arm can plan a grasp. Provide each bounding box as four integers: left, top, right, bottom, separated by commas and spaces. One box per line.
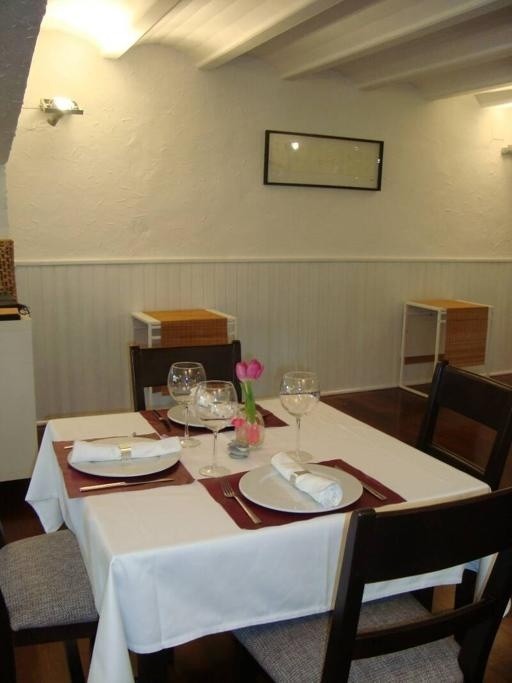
231, 412, 267, 451
191, 379, 239, 479
166, 360, 207, 450
278, 370, 321, 463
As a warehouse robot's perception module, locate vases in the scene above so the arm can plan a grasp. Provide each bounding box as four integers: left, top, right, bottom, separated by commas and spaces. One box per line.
232, 408, 266, 449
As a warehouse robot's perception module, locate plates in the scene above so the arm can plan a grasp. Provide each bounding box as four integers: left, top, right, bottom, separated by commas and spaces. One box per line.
237, 461, 365, 515
66, 436, 182, 479
166, 399, 246, 429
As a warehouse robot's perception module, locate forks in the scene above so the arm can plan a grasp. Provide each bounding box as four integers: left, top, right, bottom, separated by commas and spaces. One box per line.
218, 478, 263, 525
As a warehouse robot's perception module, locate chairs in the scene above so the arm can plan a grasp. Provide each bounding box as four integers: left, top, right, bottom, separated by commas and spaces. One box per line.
228, 488, 510, 683
0, 530, 177, 683
416, 361, 511, 492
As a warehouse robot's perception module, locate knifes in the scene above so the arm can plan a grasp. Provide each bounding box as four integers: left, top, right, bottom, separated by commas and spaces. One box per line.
79, 477, 178, 492
331, 462, 391, 502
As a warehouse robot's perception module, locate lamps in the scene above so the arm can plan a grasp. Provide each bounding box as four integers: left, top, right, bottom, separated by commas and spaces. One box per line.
38, 97, 83, 121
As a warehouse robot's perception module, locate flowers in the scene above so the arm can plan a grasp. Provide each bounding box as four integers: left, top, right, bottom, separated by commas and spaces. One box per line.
230, 360, 265, 445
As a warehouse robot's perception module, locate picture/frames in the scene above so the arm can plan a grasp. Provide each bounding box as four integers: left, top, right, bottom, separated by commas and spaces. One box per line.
263, 130, 383, 197
